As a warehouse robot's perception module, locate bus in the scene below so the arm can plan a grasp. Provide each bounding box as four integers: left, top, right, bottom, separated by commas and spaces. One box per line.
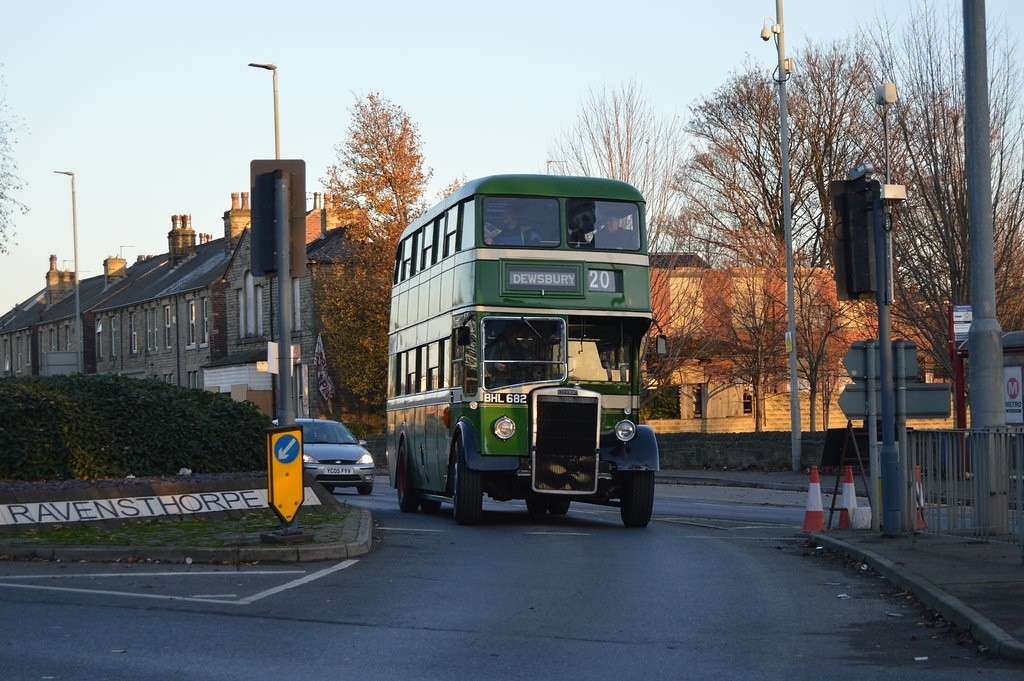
386, 176, 657, 530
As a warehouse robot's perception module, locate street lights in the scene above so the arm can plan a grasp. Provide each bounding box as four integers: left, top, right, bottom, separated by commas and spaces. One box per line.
54, 170, 83, 374
248, 62, 279, 160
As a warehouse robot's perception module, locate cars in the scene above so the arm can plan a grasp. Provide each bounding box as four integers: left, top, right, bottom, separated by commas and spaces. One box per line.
273, 419, 375, 496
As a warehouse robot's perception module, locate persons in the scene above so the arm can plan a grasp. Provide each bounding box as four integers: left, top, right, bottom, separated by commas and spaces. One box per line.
589, 212, 636, 249
485, 326, 542, 388
491, 205, 541, 246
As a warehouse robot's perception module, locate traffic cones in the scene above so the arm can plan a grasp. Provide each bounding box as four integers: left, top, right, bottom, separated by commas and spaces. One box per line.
910, 465, 925, 530
836, 465, 858, 530
802, 465, 827, 533
